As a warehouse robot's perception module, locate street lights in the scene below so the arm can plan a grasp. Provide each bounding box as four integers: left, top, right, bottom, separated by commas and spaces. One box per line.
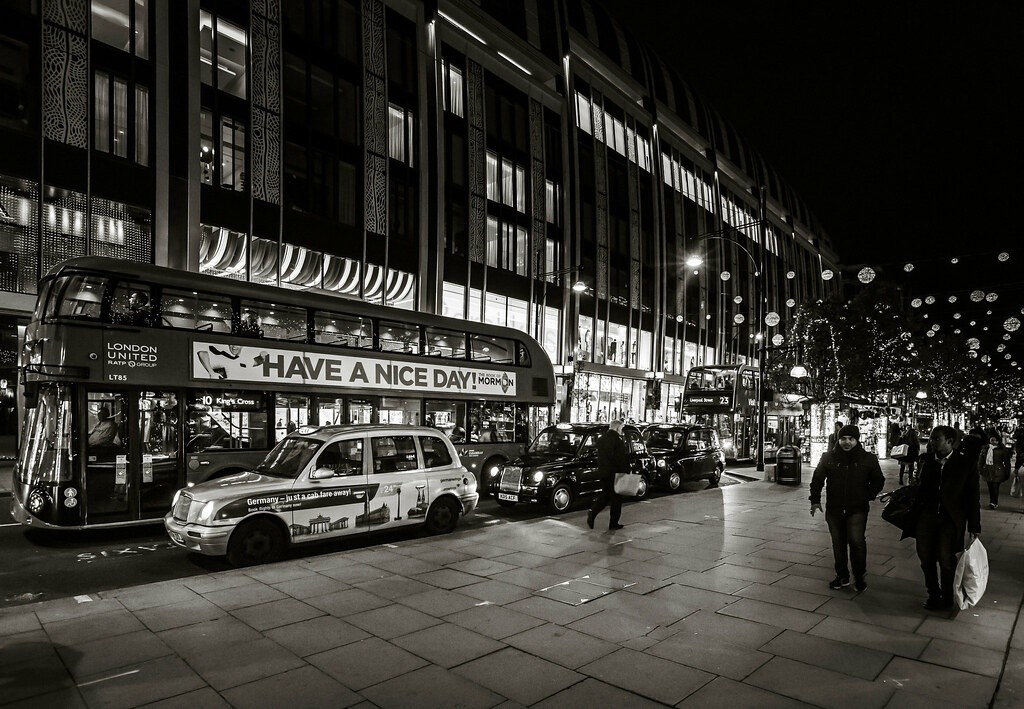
683, 235, 766, 472
756, 336, 808, 472
528, 264, 587, 339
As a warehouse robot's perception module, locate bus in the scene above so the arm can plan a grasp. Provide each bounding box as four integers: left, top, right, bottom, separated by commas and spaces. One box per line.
675, 364, 811, 463
9, 255, 557, 533
910, 401, 965, 441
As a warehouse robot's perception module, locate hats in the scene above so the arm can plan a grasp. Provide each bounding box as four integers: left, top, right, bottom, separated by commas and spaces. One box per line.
837, 425, 861, 440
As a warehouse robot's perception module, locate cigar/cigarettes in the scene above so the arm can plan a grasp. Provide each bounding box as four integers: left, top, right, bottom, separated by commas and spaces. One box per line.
810, 510, 813, 511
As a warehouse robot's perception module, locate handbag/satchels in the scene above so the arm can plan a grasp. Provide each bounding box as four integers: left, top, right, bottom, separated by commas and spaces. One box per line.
1009, 474, 1023, 497
952, 537, 989, 610
890, 444, 910, 459
880, 484, 920, 530
613, 463, 641, 496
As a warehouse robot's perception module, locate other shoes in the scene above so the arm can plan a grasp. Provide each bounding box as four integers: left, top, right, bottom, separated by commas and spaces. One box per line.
989, 503, 999, 509
922, 595, 953, 612
609, 524, 623, 530
586, 509, 595, 529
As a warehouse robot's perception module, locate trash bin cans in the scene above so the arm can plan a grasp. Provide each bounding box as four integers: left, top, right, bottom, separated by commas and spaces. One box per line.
776, 446, 802, 486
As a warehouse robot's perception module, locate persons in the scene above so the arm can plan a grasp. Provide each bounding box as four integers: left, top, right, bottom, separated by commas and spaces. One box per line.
827, 421, 1024, 509
910, 425, 981, 613
665, 351, 702, 374
579, 325, 637, 364
587, 420, 632, 530
690, 378, 735, 389
88, 407, 118, 446
587, 402, 629, 419
808, 425, 886, 592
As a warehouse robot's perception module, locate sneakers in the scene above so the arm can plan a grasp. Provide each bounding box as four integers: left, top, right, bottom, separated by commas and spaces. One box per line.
829, 576, 851, 589
852, 581, 868, 592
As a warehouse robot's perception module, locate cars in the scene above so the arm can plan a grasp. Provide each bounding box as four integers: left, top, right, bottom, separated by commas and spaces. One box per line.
487, 421, 658, 514
635, 424, 672, 448
641, 423, 727, 491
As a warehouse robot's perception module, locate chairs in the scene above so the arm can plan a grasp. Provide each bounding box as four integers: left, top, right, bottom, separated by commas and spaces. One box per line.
450, 426, 465, 443
193, 323, 514, 365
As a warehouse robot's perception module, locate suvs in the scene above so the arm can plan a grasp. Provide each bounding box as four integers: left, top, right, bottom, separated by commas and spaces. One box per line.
164, 424, 481, 569
